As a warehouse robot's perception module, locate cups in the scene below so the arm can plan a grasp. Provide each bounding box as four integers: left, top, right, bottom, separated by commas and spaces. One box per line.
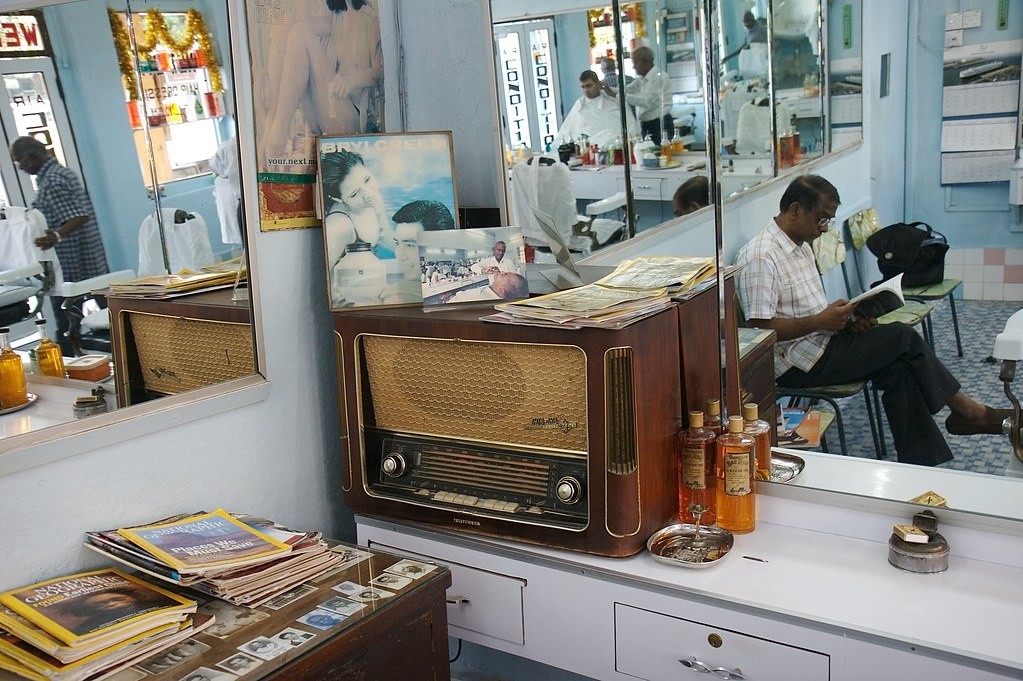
139, 59, 157, 72
195, 50, 206, 67
621, 7, 634, 22
155, 52, 169, 71
605, 12, 611, 25
606, 49, 613, 59
642, 156, 668, 168
126, 101, 140, 128
624, 52, 630, 58
205, 92, 218, 117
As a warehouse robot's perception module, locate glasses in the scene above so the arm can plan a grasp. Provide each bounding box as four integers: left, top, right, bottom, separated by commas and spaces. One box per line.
802, 202, 835, 231
14, 151, 31, 167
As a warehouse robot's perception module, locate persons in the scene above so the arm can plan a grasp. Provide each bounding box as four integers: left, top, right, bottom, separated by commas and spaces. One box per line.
721, 10, 765, 65
600, 56, 637, 118
421, 242, 529, 301
671, 174, 711, 216
602, 48, 676, 144
732, 173, 1023, 470
553, 70, 638, 153
257, 0, 385, 166
317, 151, 397, 302
377, 202, 455, 305
10, 137, 111, 284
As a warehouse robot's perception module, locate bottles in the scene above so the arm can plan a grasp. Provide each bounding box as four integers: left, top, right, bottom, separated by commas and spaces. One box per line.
675, 411, 716, 526
703, 398, 729, 436
557, 127, 684, 166
179, 53, 195, 67
777, 129, 800, 168
742, 403, 770, 482
803, 71, 818, 96
334, 243, 386, 305
716, 415, 756, 534
506, 143, 531, 167
0, 328, 26, 409
191, 88, 205, 120
35, 318, 66, 379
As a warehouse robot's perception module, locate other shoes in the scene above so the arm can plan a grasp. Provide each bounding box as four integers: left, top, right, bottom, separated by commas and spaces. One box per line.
945, 406, 1023, 436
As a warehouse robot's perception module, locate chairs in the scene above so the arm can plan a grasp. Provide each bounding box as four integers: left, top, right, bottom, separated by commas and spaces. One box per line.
734, 97, 798, 155
733, 293, 887, 460
845, 207, 964, 359
809, 228, 936, 354
208, 135, 245, 246
60, 207, 215, 362
0, 205, 65, 351
671, 104, 698, 150
510, 156, 640, 264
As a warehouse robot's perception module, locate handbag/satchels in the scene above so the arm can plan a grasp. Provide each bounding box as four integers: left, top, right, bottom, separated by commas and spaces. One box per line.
866, 222, 950, 289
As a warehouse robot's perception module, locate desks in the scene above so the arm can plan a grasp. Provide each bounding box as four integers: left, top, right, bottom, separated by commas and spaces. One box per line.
354, 493, 1023, 681
569, 155, 771, 203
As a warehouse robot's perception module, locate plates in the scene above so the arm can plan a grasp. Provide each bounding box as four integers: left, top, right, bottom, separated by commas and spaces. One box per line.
639, 161, 682, 170
0, 392, 38, 414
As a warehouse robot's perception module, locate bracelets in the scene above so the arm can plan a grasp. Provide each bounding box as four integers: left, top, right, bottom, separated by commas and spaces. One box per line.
53, 231, 62, 242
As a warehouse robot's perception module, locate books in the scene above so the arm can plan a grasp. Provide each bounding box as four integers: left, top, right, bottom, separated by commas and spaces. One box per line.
0, 567, 219, 681
82, 511, 350, 608
847, 273, 909, 323
479, 280, 675, 330
593, 254, 744, 300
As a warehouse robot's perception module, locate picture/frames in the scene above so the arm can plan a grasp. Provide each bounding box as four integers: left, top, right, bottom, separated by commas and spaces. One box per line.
314, 129, 460, 312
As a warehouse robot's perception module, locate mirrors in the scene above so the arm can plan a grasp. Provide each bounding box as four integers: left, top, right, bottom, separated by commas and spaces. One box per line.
489, 1, 866, 265
0, 0, 246, 363
0, 0, 272, 478
482, 0, 717, 268
708, 0, 1023, 523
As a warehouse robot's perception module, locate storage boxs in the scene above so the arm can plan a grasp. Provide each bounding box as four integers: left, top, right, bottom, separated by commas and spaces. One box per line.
892, 524, 930, 545
908, 490, 947, 506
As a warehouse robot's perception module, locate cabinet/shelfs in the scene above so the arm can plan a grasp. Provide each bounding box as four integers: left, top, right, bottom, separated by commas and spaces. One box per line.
132, 65, 229, 136
0, 539, 453, 681
720, 327, 779, 447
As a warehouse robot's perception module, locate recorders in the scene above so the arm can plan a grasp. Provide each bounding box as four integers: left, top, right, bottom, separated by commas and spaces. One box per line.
331, 302, 681, 557
524, 263, 741, 438
107, 288, 258, 411
